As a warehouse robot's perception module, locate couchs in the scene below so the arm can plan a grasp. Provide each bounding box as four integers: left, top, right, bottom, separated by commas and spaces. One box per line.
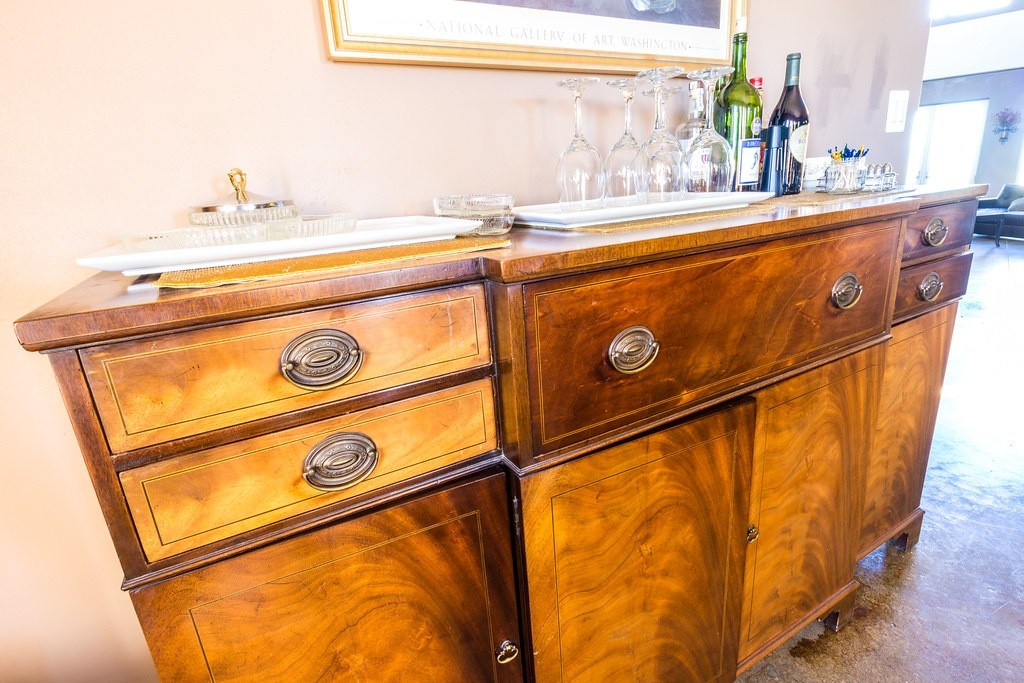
973, 184, 1024, 247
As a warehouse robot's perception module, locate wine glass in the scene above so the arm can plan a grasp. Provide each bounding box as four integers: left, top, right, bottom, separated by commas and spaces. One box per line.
557, 76, 606, 212
641, 83, 683, 201
603, 78, 645, 209
685, 65, 736, 201
634, 65, 687, 206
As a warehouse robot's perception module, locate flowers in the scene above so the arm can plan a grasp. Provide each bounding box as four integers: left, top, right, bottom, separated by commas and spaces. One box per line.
995, 109, 1022, 127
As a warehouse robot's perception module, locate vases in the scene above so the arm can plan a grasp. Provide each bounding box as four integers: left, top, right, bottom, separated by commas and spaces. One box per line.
997, 124, 1011, 142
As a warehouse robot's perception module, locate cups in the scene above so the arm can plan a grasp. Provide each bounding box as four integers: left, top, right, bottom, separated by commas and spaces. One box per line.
431, 192, 515, 235
760, 124, 789, 197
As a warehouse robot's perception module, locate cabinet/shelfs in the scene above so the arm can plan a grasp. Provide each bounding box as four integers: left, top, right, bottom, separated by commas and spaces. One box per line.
11, 174, 988, 673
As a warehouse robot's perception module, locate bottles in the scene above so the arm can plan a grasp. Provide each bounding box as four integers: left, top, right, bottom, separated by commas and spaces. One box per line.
714, 32, 768, 192
824, 156, 866, 195
767, 53, 809, 194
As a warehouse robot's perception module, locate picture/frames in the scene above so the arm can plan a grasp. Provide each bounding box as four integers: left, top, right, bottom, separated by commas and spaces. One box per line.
318, 0, 748, 90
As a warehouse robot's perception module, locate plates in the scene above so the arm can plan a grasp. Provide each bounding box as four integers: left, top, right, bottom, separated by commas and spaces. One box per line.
74, 210, 484, 277
510, 191, 775, 229
